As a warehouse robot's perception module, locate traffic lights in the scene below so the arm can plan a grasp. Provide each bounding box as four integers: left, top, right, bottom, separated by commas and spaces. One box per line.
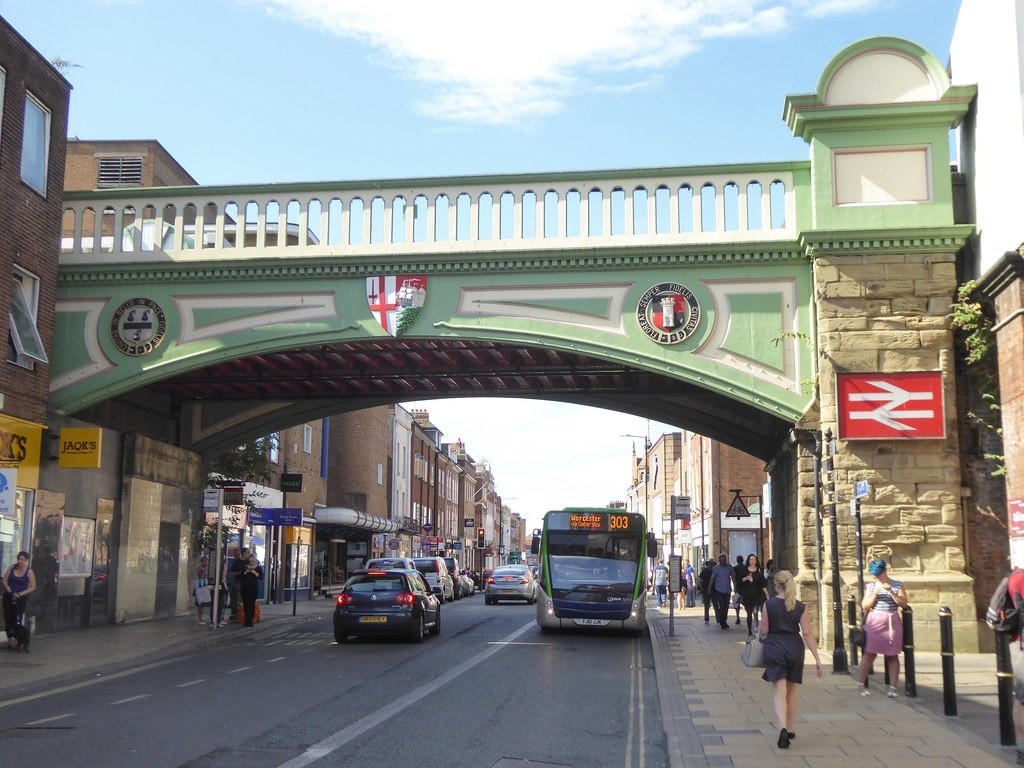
477, 529, 485, 549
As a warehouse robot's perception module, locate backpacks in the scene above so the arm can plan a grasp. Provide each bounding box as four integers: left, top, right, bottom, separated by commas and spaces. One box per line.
985, 569, 1024, 635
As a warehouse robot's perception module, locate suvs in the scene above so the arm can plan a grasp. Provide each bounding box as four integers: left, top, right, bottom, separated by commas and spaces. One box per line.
413, 556, 455, 604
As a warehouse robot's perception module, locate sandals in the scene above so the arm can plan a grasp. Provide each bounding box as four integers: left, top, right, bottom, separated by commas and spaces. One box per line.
887, 686, 897, 697
858, 683, 871, 696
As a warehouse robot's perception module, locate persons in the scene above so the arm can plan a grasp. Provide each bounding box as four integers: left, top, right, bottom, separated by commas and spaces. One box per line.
652, 558, 698, 609
192, 546, 264, 627
1, 551, 36, 651
856, 560, 908, 698
700, 554, 776, 636
1007, 569, 1024, 767
759, 570, 823, 748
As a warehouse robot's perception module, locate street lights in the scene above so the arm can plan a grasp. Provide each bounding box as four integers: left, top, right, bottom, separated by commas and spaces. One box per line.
622, 434, 648, 531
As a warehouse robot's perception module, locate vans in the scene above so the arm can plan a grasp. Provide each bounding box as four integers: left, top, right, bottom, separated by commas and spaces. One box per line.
363, 558, 416, 571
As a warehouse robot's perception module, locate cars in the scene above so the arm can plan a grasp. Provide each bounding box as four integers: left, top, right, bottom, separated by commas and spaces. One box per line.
443, 556, 475, 600
530, 565, 539, 580
483, 565, 537, 605
333, 567, 442, 643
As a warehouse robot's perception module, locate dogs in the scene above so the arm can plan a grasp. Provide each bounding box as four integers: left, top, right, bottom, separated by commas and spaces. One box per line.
5, 624, 31, 653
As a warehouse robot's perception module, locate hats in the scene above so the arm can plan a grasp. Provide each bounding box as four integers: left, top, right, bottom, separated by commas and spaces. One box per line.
869, 560, 886, 576
201, 556, 209, 562
705, 558, 717, 566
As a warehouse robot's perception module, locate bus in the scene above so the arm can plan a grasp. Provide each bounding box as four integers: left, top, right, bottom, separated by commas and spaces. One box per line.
531, 507, 657, 635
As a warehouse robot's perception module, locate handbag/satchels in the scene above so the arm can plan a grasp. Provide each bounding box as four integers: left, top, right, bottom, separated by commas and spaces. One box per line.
733, 593, 741, 609
196, 578, 211, 604
742, 627, 765, 667
849, 628, 866, 648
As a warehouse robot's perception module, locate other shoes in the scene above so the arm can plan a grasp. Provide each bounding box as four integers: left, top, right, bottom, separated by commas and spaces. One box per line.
11, 639, 18, 650
662, 600, 666, 607
198, 621, 227, 628
0, 638, 12, 648
789, 732, 795, 739
778, 729, 790, 748
736, 619, 740, 625
721, 622, 729, 629
